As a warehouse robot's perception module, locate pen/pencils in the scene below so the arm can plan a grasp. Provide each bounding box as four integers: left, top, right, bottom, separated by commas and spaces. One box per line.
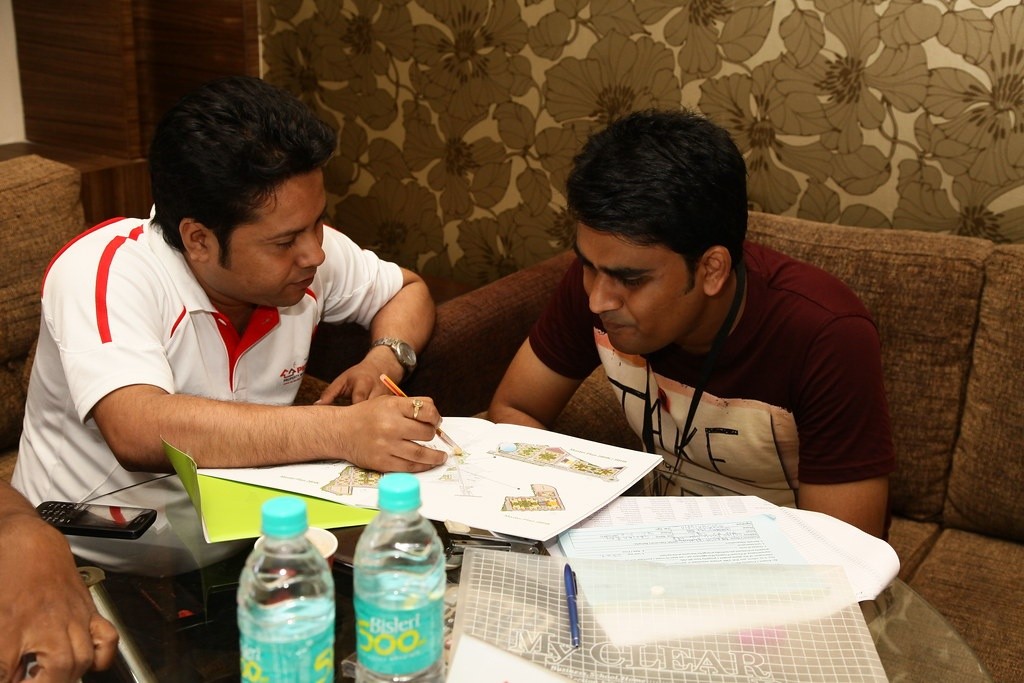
564, 563, 580, 651
379, 373, 464, 457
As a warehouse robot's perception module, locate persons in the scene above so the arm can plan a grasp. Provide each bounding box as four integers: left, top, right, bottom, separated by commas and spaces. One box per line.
488, 106, 889, 540
10, 74, 449, 683
0, 478, 120, 683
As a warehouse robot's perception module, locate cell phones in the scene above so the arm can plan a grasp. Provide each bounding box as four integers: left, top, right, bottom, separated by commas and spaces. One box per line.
37, 500, 158, 540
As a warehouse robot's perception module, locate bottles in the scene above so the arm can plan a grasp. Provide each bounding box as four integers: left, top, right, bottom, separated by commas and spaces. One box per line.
237, 496, 335, 683
354, 473, 447, 682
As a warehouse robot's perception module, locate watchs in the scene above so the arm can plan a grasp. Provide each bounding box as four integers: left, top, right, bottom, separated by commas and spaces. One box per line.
370, 338, 417, 376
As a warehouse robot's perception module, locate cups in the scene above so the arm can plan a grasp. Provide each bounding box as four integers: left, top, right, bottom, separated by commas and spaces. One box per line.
255, 527, 338, 605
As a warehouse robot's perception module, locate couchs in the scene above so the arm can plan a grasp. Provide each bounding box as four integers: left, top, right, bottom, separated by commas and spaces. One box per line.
398, 209, 1024, 683
0, 155, 332, 487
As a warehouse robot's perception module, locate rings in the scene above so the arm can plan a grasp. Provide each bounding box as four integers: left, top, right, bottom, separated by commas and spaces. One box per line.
413, 399, 423, 419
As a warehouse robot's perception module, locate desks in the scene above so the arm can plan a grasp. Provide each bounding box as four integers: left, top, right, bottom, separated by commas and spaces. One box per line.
63, 469, 993, 683
